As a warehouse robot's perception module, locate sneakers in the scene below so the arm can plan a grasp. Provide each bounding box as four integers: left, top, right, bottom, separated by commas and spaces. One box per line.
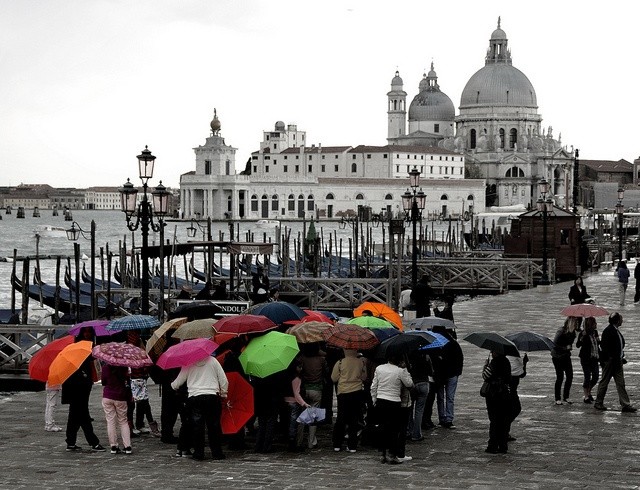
111, 446, 119, 454
397, 456, 413, 463
445, 421, 456, 429
346, 446, 357, 453
44, 423, 48, 431
182, 449, 192, 457
66, 446, 82, 451
334, 447, 340, 451
175, 450, 181, 457
123, 446, 133, 454
47, 425, 62, 432
91, 444, 107, 451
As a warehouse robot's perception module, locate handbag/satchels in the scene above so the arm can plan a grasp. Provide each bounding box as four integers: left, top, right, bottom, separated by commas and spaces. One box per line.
273, 382, 297, 417
480, 366, 507, 401
371, 400, 392, 438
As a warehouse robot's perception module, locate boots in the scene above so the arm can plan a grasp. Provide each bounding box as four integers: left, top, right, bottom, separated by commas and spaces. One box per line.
128, 421, 140, 438
148, 420, 161, 438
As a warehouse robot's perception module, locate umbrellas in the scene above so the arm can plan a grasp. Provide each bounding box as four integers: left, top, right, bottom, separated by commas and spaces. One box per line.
344, 316, 396, 331
104, 314, 161, 331
353, 301, 403, 331
92, 342, 154, 368
411, 330, 450, 349
68, 320, 123, 337
407, 317, 458, 329
377, 331, 437, 353
47, 340, 93, 387
212, 315, 279, 335
29, 334, 74, 381
239, 302, 310, 325
156, 338, 219, 370
321, 324, 380, 350
503, 330, 556, 351
560, 303, 611, 318
218, 371, 254, 434
285, 321, 333, 343
238, 330, 300, 379
318, 311, 338, 321
463, 332, 521, 357
284, 310, 335, 326
171, 318, 218, 339
145, 317, 188, 356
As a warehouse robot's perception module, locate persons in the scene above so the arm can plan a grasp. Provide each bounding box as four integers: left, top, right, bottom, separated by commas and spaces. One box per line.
411, 274, 431, 331
370, 354, 413, 465
498, 347, 529, 441
177, 285, 191, 299
330, 348, 366, 453
161, 337, 187, 445
218, 336, 304, 457
430, 295, 456, 321
570, 276, 595, 307
633, 261, 640, 305
297, 341, 332, 449
552, 317, 584, 405
480, 350, 513, 454
594, 316, 638, 412
73, 328, 100, 343
617, 261, 630, 307
266, 289, 279, 303
395, 361, 413, 462
400, 286, 412, 310
408, 349, 433, 442
578, 318, 601, 407
171, 355, 229, 461
212, 280, 228, 301
195, 282, 212, 299
252, 267, 269, 304
63, 361, 106, 454
436, 328, 464, 430
101, 365, 134, 454
44, 379, 65, 433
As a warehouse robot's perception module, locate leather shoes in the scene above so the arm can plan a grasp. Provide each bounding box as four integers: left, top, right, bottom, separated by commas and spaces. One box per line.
594, 402, 607, 411
621, 406, 637, 414
